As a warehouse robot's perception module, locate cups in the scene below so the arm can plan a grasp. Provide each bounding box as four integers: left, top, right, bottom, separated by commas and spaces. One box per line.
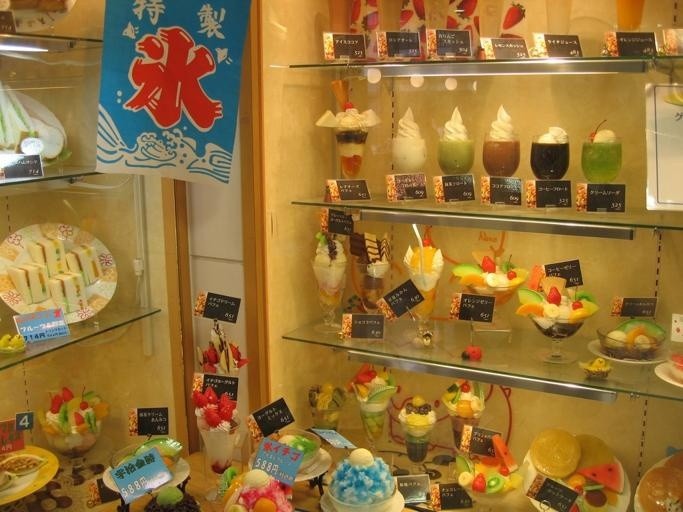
614, 1, 645, 34
308, 404, 342, 432
547, 0, 574, 35
397, 414, 437, 477
349, 256, 393, 316
353, 397, 389, 457
476, 1, 504, 50
402, 260, 446, 344
334, 124, 623, 185
449, 413, 481, 456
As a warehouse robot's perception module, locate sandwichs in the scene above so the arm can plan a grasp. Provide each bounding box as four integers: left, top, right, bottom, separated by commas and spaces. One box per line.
8, 236, 104, 315
0, 81, 64, 162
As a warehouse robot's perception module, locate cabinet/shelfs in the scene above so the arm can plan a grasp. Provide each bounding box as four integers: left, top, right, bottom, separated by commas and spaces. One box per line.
240, 0, 681, 511
0, 0, 200, 458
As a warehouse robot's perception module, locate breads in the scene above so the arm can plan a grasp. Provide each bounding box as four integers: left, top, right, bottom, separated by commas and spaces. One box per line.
0, 0, 75, 34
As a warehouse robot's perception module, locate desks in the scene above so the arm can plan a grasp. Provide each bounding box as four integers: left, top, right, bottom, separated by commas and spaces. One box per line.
110, 451, 416, 511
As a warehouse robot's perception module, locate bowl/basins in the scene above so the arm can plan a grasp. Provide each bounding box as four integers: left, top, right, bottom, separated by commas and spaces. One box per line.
265, 426, 322, 470
595, 324, 668, 359
324, 462, 399, 512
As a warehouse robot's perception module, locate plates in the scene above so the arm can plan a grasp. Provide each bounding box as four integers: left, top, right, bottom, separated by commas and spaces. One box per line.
0, 445, 61, 506
653, 358, 683, 388
318, 487, 406, 512
101, 452, 192, 497
0, 221, 119, 327
0, 88, 68, 170
247, 445, 334, 484
521, 442, 633, 511
632, 454, 677, 512
587, 336, 671, 365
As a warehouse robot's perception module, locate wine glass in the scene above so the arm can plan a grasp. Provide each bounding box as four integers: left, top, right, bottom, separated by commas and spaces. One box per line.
311, 257, 345, 338
37, 415, 105, 488
456, 269, 529, 331
531, 313, 586, 366
196, 418, 245, 505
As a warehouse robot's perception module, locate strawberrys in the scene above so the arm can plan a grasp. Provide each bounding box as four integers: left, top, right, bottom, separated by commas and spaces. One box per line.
344, 0, 526, 61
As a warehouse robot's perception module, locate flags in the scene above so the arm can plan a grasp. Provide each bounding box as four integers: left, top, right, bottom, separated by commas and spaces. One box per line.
95, 1, 250, 184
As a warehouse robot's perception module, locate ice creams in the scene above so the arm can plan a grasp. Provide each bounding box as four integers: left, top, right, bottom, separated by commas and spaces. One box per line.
391, 106, 428, 173
315, 80, 381, 180
483, 104, 520, 178
36, 385, 109, 456
349, 231, 393, 316
402, 224, 446, 324
530, 126, 570, 181
581, 119, 624, 185
514, 263, 601, 341
595, 296, 668, 360
451, 248, 529, 307
111, 323, 683, 512
437, 105, 475, 175
311, 232, 349, 318
